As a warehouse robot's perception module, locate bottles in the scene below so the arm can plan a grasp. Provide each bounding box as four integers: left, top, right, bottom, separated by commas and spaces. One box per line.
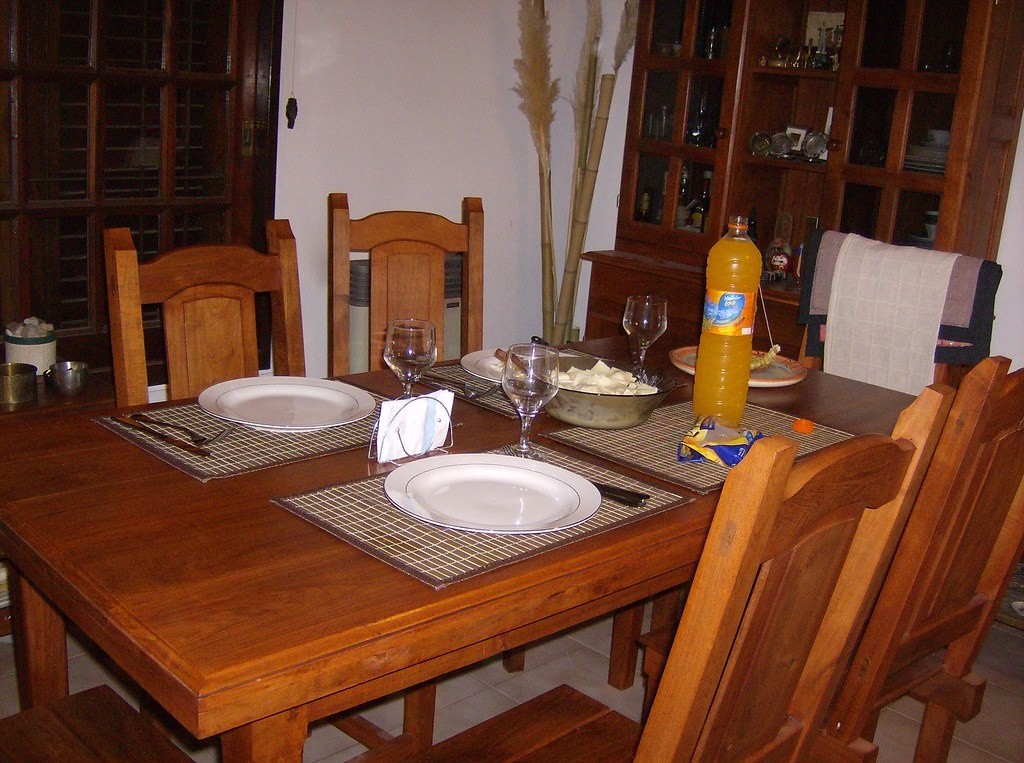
691, 216, 765, 426
632, 0, 736, 231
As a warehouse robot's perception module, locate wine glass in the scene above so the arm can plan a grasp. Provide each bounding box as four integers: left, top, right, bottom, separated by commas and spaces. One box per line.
496, 343, 559, 459
622, 295, 668, 363
382, 318, 438, 399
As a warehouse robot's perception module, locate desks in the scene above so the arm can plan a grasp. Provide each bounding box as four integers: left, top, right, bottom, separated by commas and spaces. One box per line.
1, 331, 917, 763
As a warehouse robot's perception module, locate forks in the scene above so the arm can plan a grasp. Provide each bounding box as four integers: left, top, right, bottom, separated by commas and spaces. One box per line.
129, 412, 236, 446
421, 374, 501, 396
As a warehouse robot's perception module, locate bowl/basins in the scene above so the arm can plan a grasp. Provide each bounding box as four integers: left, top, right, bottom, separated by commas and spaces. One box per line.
522, 356, 677, 430
43, 362, 89, 395
927, 129, 950, 147
0, 362, 38, 405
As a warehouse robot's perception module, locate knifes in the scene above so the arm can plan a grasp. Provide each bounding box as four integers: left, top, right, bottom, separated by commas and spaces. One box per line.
111, 414, 212, 456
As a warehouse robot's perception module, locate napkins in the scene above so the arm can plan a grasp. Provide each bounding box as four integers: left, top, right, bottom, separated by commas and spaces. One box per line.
376, 389, 454, 463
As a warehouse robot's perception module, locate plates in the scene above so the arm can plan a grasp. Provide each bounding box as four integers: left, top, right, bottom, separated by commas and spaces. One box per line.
900, 143, 947, 174
460, 348, 504, 383
198, 376, 377, 433
668, 344, 811, 387
383, 453, 603, 535
751, 130, 828, 159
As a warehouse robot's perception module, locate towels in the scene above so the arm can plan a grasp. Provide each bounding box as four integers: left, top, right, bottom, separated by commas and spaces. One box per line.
824, 233, 960, 398
798, 221, 1005, 368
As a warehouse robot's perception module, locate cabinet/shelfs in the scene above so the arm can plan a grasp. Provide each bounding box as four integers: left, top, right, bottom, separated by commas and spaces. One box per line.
580, 0, 1024, 388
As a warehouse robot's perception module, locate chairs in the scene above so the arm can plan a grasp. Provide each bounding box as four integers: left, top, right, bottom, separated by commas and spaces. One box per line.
0, 193, 1024, 763
